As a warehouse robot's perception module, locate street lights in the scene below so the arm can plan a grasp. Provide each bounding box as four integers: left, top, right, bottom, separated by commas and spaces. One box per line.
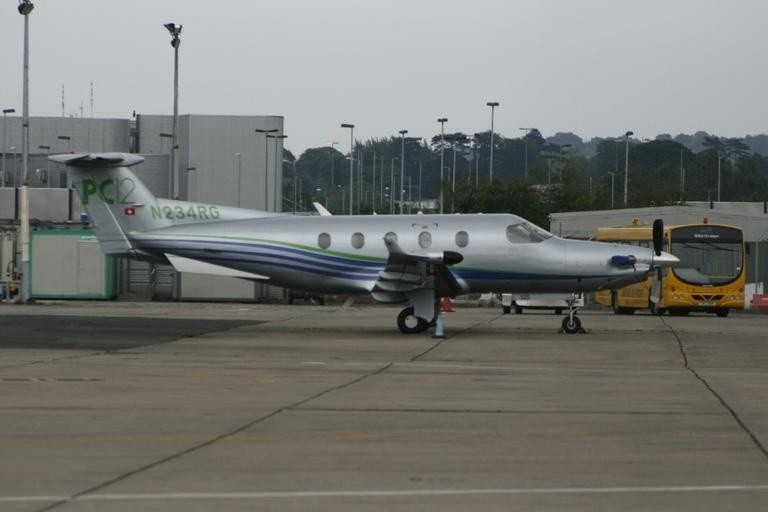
332, 118, 478, 215
542, 142, 572, 184
2, 109, 69, 189
255, 129, 297, 215
519, 128, 534, 182
165, 24, 183, 199
608, 130, 633, 210
18, 2, 33, 302
158, 133, 195, 202
487, 103, 499, 189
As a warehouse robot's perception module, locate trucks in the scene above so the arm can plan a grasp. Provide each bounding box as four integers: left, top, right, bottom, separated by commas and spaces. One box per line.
501, 292, 584, 315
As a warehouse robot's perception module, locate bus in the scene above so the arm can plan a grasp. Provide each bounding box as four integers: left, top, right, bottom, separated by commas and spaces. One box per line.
596, 220, 750, 316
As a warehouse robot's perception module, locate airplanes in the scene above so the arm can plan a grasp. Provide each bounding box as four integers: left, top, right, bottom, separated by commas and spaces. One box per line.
48, 151, 681, 333
